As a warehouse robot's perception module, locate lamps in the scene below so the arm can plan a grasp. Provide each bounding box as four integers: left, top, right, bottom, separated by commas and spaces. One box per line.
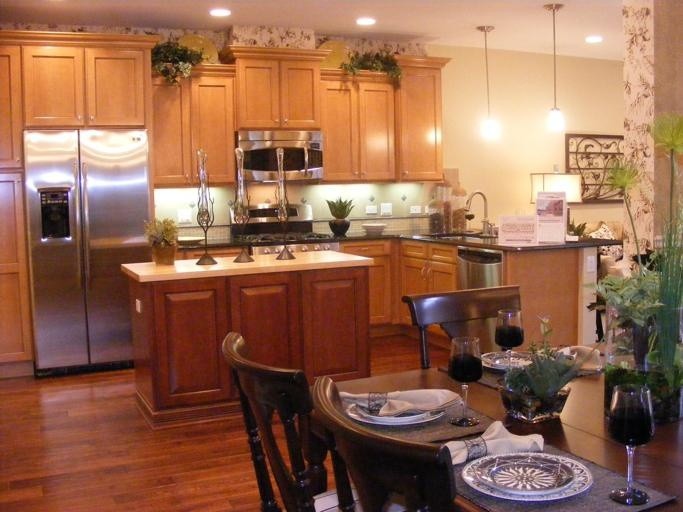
192, 144, 219, 266
543, 3, 570, 136
227, 147, 255, 264
528, 162, 585, 243
274, 146, 296, 261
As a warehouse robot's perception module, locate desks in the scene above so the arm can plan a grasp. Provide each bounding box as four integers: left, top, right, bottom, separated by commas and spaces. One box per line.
119, 249, 375, 432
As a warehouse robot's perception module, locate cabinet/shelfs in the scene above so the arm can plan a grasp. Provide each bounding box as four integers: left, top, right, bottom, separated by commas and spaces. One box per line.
398, 238, 459, 351
20, 31, 162, 130
147, 63, 237, 187
216, 46, 334, 134
0, 29, 37, 379
396, 54, 453, 183
338, 239, 398, 340
303, 67, 395, 185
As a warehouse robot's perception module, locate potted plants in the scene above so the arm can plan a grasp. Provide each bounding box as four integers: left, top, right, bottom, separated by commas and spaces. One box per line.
323, 197, 354, 237
577, 106, 682, 446
496, 310, 607, 426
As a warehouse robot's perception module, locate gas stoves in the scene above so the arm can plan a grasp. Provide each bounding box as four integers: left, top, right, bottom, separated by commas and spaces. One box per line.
237, 231, 340, 254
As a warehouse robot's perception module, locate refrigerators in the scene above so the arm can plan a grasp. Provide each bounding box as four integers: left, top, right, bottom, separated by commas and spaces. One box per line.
23, 128, 160, 379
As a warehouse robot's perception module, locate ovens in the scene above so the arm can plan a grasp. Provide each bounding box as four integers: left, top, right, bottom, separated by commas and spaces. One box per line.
454, 245, 504, 290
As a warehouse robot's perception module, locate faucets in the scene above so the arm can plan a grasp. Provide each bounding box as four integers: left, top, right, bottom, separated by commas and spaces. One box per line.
464, 191, 490, 238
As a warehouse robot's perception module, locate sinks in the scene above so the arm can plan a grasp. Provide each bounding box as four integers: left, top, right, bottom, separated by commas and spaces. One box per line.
432, 232, 484, 246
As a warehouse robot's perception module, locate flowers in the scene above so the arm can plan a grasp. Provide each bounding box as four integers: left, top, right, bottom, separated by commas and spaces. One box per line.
142, 215, 179, 248
148, 31, 209, 85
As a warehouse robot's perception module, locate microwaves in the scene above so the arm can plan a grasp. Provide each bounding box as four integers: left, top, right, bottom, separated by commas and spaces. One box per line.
236, 130, 324, 181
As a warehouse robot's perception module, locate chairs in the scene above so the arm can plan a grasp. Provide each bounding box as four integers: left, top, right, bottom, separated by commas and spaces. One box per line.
219, 327, 364, 511
399, 282, 523, 368
311, 370, 459, 512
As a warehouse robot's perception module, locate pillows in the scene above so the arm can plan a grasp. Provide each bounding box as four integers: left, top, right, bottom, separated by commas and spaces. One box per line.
585, 220, 624, 262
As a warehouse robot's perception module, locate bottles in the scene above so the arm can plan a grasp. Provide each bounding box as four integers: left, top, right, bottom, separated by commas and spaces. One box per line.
425, 175, 469, 234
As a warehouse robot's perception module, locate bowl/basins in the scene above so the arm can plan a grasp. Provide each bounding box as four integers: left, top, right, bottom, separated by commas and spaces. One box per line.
360, 222, 387, 235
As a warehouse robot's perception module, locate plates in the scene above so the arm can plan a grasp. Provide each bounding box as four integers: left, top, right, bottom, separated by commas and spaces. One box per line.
478, 349, 537, 372
460, 451, 594, 503
176, 33, 219, 64
343, 401, 444, 425
176, 237, 204, 246
315, 39, 357, 70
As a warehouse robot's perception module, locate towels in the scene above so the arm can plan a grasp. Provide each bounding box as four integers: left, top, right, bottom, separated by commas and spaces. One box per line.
553, 343, 604, 378
439, 418, 547, 465
338, 385, 462, 418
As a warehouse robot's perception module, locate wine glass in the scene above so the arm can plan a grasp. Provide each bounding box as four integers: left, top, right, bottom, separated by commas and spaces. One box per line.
604, 383, 657, 506
447, 333, 484, 427
493, 304, 525, 385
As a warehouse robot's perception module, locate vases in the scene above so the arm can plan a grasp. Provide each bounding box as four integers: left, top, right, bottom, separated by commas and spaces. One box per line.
152, 243, 176, 266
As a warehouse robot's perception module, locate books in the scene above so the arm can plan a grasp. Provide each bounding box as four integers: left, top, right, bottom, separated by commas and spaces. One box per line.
536, 198, 565, 245
538, 192, 566, 239
499, 215, 537, 245
